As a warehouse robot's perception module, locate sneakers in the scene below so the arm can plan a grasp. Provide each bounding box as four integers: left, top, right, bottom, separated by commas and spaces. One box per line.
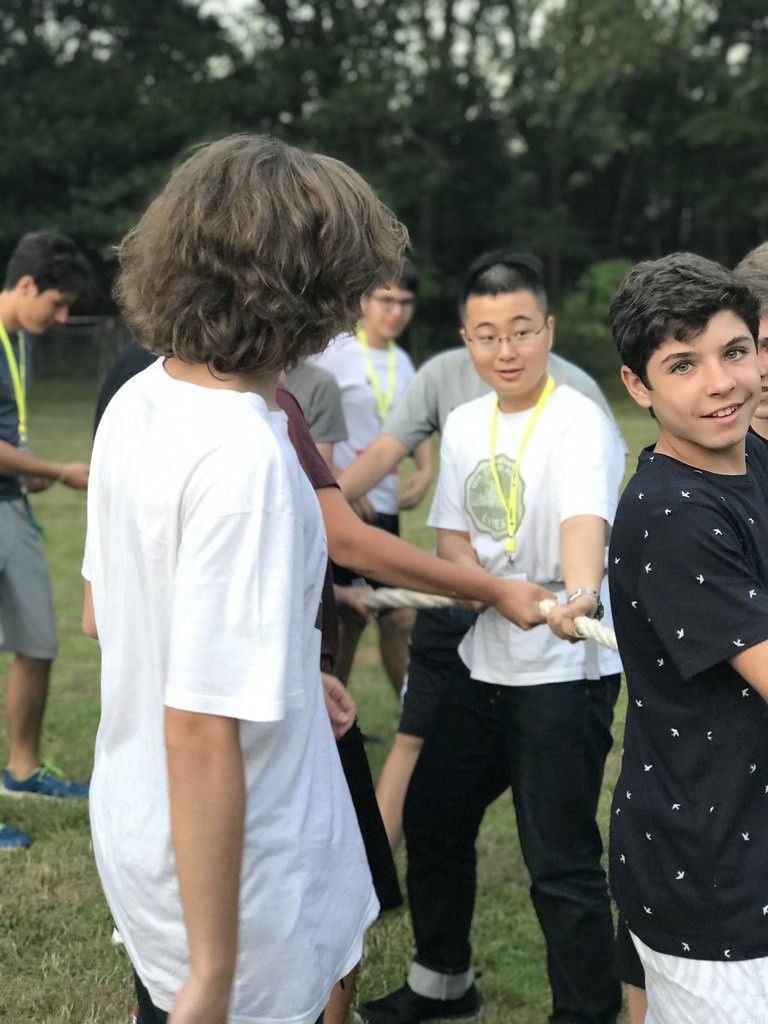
0, 820, 30, 849
353, 979, 482, 1023
1, 761, 91, 803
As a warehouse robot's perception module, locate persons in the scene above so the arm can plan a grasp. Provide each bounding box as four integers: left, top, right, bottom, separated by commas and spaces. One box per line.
0, 231, 88, 851
616, 244, 767, 1024
359, 259, 625, 1024
281, 353, 348, 469
81, 136, 408, 1024
607, 251, 767, 1023
306, 258, 431, 705
274, 364, 558, 1024
337, 246, 607, 854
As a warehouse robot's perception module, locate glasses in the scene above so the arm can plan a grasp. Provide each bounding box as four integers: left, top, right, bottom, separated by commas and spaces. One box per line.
367, 293, 416, 313
460, 315, 548, 352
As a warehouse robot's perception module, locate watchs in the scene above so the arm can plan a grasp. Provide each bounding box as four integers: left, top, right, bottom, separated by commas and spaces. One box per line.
567, 589, 604, 621
